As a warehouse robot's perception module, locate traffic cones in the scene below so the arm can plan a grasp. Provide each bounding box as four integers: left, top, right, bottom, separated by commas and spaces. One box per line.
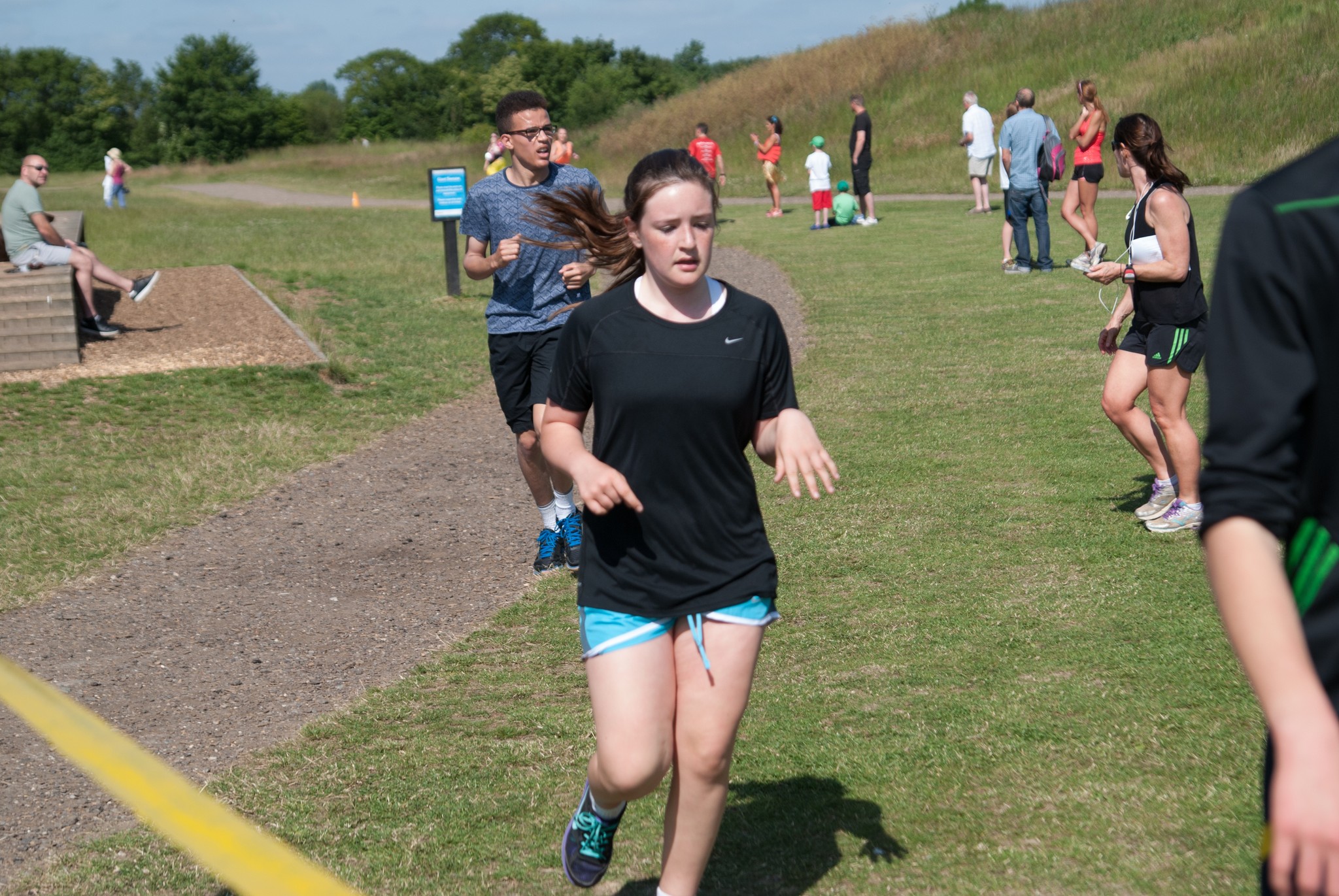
352, 191, 361, 209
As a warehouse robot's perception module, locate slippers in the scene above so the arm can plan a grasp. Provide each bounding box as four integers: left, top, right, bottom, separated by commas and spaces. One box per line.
823, 224, 830, 228
810, 223, 821, 229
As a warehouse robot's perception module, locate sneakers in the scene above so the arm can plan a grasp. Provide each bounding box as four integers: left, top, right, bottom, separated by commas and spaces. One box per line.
1144, 498, 1204, 533
533, 527, 564, 575
80, 315, 119, 336
128, 271, 159, 303
1065, 251, 1091, 272
555, 505, 583, 569
1087, 241, 1107, 267
561, 781, 628, 888
1002, 258, 1015, 270
862, 217, 878, 225
1134, 478, 1179, 521
856, 214, 865, 224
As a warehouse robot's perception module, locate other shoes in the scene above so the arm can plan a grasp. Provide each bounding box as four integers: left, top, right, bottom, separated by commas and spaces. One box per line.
984, 207, 991, 213
767, 208, 782, 217
1005, 263, 1025, 274
967, 206, 984, 215
1035, 266, 1052, 272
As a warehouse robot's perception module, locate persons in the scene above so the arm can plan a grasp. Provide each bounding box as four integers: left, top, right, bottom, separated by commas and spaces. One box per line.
998, 87, 1060, 273
999, 101, 1037, 272
459, 90, 611, 576
847, 93, 879, 226
483, 133, 507, 177
1060, 80, 1109, 269
510, 149, 841, 896
688, 123, 726, 186
548, 128, 580, 164
1082, 113, 1209, 534
104, 148, 132, 209
1, 155, 162, 336
1197, 134, 1339, 896
749, 115, 785, 217
804, 136, 833, 230
958, 90, 996, 215
827, 180, 861, 226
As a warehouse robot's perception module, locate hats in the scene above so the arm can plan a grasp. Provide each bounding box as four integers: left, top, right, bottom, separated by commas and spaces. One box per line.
809, 136, 824, 146
838, 181, 848, 191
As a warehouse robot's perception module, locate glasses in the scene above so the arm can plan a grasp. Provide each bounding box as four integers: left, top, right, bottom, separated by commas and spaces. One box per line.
506, 125, 557, 137
27, 164, 50, 172
1111, 141, 1117, 151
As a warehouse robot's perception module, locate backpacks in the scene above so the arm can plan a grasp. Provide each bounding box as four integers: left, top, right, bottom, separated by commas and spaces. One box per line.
1035, 114, 1065, 182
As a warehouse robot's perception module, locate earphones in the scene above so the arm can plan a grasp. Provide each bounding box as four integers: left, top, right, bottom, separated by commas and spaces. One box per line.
1126, 205, 1135, 221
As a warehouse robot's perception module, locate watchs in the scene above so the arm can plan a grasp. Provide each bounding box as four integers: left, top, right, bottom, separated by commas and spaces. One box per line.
1121, 263, 1135, 284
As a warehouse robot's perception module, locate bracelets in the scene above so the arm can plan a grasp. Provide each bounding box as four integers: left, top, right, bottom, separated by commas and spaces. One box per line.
720, 174, 725, 175
754, 140, 758, 144
